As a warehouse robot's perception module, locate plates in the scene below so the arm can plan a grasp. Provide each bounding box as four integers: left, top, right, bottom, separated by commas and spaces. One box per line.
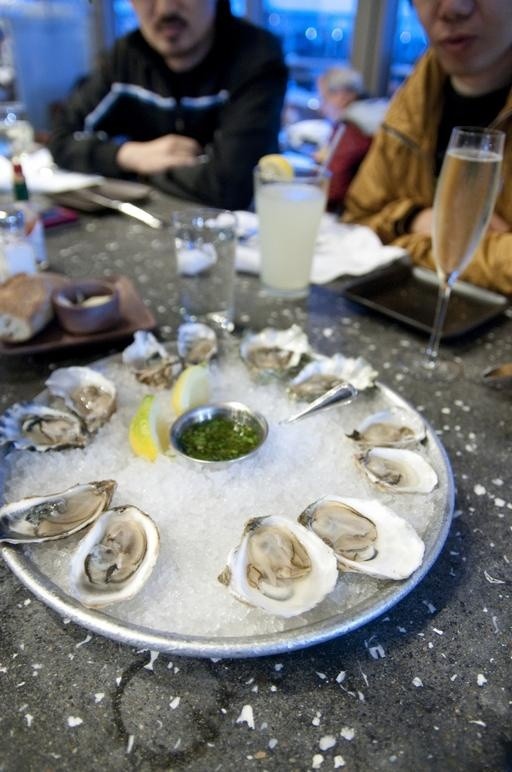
0, 336, 458, 659
170, 403, 268, 465
345, 256, 507, 342
0, 274, 157, 358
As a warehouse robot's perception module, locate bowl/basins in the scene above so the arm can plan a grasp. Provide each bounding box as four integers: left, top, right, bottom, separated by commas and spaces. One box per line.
49, 280, 124, 336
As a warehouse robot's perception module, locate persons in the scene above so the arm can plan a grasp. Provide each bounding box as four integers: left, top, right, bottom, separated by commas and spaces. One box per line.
315, 67, 390, 213
45, 0, 290, 212
340, 0, 512, 297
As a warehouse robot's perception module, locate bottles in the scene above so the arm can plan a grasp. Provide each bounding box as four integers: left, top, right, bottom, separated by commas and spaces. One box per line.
5, 211, 37, 274
12, 163, 48, 271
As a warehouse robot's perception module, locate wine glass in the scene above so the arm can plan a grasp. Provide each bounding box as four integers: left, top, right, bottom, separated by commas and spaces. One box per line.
395, 124, 510, 380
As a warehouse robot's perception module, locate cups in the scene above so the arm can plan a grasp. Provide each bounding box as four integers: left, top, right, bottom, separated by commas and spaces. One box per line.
171, 206, 239, 333
249, 159, 334, 302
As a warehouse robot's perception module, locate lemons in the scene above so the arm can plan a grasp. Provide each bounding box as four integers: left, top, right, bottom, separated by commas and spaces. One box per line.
129, 394, 161, 461
172, 359, 210, 418
256, 154, 294, 181
